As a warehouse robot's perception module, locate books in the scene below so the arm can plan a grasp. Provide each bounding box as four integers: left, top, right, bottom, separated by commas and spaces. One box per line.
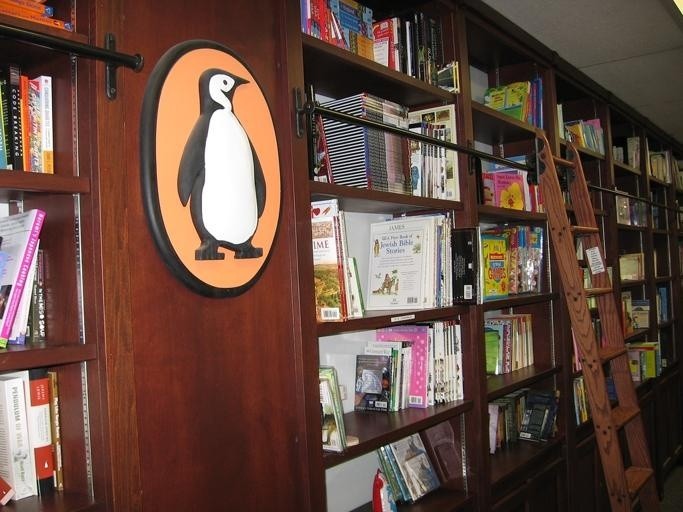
301, 1, 681, 510
0, 0, 72, 510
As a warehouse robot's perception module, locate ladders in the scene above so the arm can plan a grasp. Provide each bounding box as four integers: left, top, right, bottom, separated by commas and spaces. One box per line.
536, 132, 660, 512
